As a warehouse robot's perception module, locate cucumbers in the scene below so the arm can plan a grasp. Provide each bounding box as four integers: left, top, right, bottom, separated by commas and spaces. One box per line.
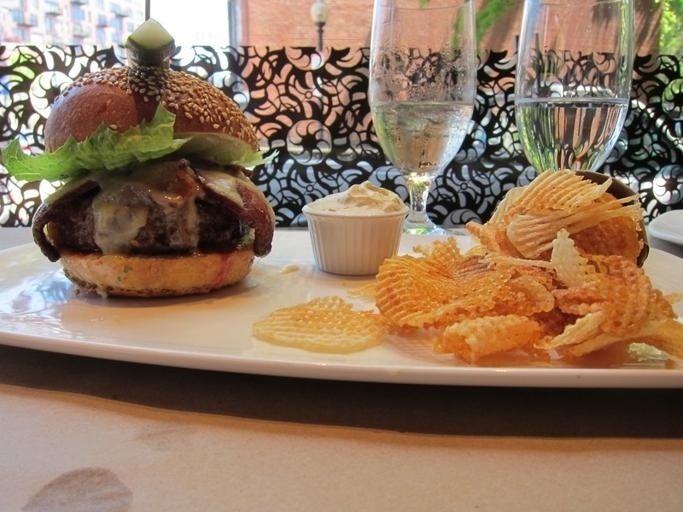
126, 19, 175, 65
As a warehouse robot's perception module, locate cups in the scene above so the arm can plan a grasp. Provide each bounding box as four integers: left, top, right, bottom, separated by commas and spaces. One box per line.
516, 0, 633, 177
301, 202, 408, 275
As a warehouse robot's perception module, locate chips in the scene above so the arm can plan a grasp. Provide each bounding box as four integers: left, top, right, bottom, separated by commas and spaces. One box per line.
253, 170, 683, 360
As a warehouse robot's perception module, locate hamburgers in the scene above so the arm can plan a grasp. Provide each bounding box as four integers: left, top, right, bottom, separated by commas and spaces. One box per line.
32, 66, 274, 298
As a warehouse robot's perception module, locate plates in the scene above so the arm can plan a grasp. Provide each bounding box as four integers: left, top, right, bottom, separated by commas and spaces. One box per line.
2, 228, 682, 393
648, 209, 681, 246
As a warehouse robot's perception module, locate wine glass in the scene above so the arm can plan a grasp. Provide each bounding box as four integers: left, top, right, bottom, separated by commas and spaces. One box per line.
371, 1, 478, 233
